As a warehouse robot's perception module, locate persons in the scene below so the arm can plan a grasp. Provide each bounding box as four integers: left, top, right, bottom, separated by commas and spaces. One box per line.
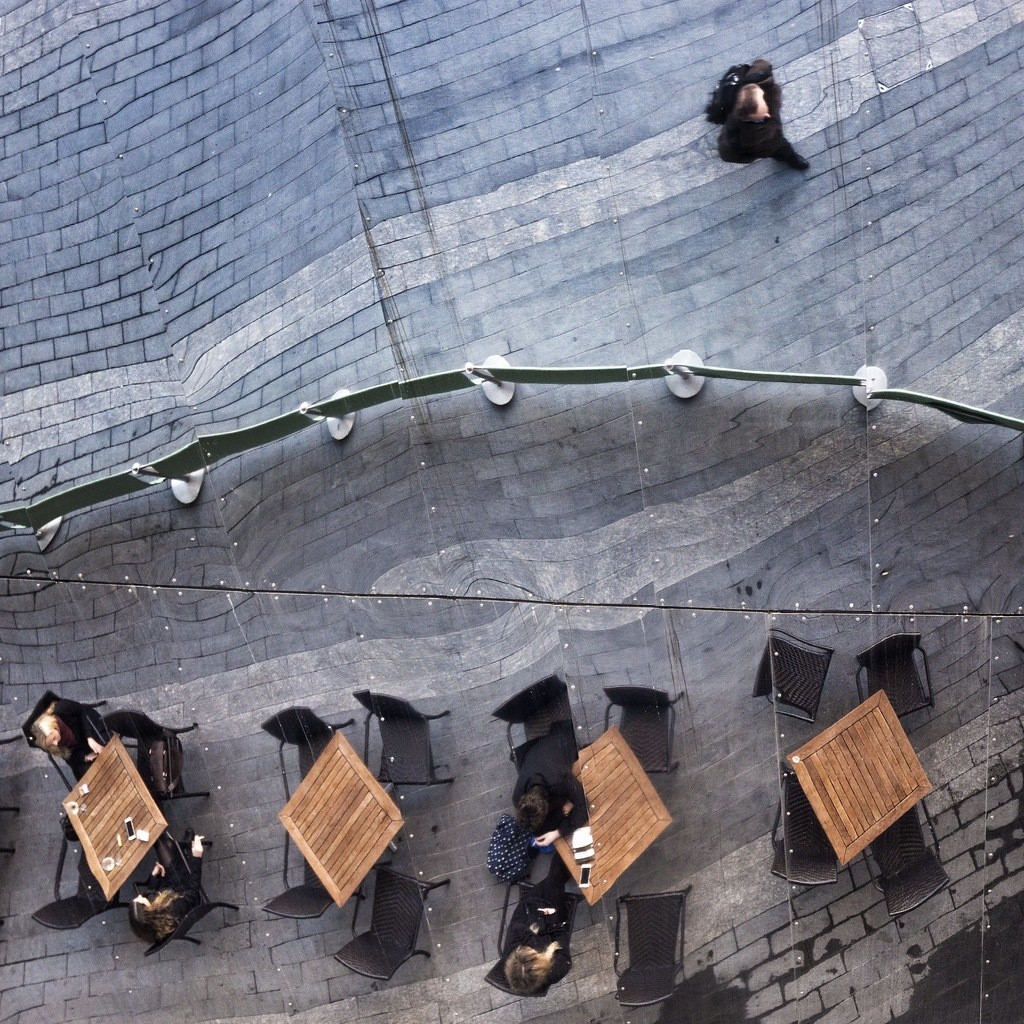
127, 823, 206, 944
501, 844, 578, 995
704, 59, 810, 171
509, 729, 588, 847
30, 696, 113, 783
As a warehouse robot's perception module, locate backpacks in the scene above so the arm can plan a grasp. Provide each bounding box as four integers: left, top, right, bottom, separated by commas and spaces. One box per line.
60, 815, 79, 843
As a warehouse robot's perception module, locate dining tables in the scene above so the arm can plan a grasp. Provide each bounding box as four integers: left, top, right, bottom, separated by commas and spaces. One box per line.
554, 725, 673, 905
61, 735, 168, 903
786, 689, 933, 866
277, 730, 405, 908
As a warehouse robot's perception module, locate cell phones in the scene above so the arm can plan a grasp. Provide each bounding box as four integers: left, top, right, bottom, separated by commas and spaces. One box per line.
124, 816, 137, 841
579, 864, 591, 888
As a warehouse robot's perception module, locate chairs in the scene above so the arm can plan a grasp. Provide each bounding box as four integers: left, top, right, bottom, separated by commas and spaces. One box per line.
353, 690, 453, 785
262, 829, 366, 919
262, 706, 354, 803
30, 834, 129, 930
484, 875, 585, 998
753, 628, 834, 723
857, 632, 934, 717
21, 691, 113, 793
614, 886, 691, 1007
492, 675, 571, 773
334, 861, 450, 981
771, 763, 839, 886
144, 849, 242, 956
862, 797, 950, 916
604, 685, 684, 775
99, 708, 210, 801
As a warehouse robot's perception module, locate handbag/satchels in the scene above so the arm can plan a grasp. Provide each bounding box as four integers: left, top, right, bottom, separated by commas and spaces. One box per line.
487, 815, 533, 882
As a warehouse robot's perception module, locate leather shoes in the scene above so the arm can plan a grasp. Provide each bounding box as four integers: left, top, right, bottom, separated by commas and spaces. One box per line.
184, 828, 193, 841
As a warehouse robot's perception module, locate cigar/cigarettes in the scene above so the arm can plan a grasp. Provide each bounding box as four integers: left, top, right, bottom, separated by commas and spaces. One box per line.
532, 840, 536, 847
197, 835, 205, 841
